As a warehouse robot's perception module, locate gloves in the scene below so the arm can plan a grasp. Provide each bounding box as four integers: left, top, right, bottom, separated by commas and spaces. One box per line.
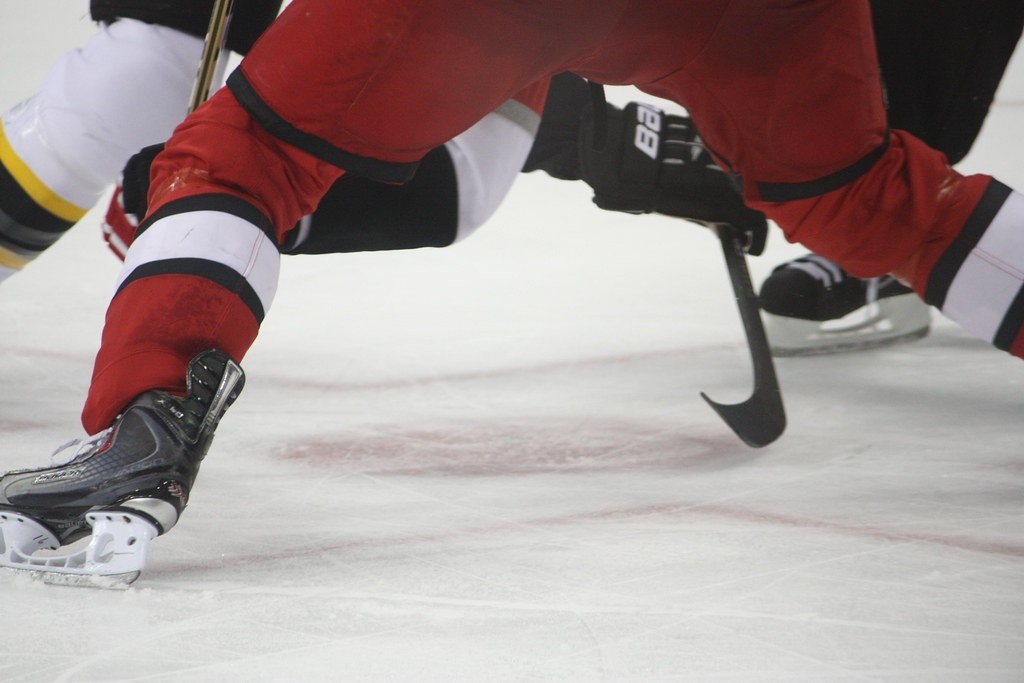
575, 77, 768, 256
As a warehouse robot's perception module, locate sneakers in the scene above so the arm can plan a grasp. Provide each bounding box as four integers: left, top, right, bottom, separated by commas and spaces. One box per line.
756, 252, 932, 359
0, 347, 246, 592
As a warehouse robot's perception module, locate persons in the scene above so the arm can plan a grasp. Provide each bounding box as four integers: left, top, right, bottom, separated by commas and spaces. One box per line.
758, 0, 1024, 353
1, 1, 1023, 588
0, 0, 769, 279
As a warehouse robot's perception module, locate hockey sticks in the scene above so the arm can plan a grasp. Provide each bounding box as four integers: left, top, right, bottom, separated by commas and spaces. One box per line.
695, 226, 788, 447
182, 0, 229, 122
